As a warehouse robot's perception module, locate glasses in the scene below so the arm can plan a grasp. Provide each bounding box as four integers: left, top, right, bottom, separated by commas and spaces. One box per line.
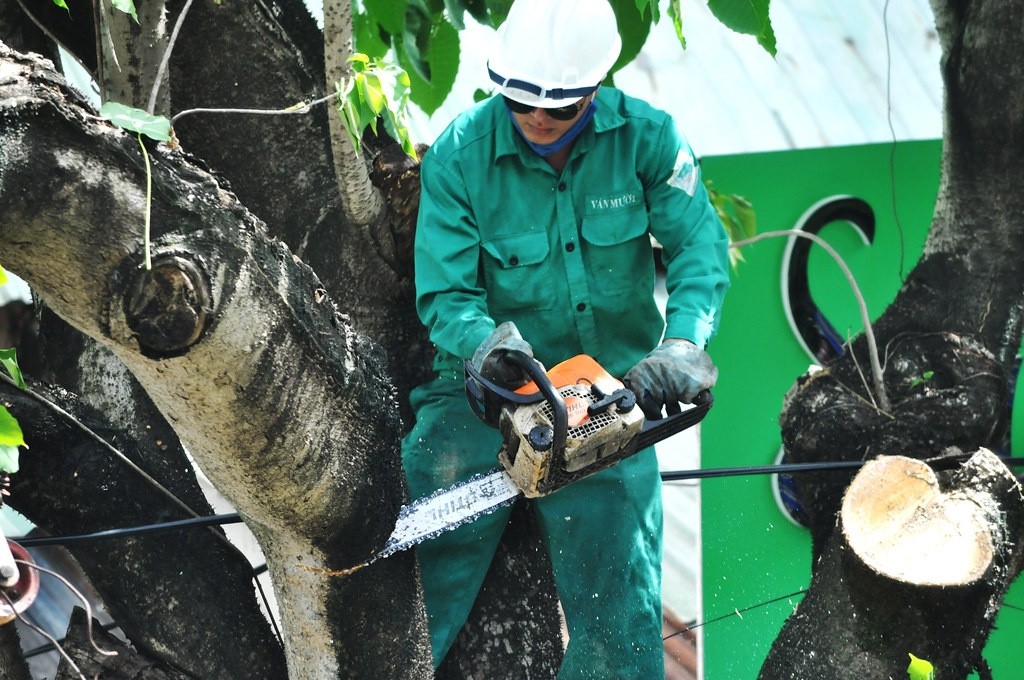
502, 94, 588, 121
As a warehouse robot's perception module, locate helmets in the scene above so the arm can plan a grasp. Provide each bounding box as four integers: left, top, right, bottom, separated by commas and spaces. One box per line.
486, 0, 624, 108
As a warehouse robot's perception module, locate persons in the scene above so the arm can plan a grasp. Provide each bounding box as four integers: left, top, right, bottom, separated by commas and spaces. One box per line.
398, 0, 731, 680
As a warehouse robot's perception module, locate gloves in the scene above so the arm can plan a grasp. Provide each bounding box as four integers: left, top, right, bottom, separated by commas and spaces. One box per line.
469, 319, 547, 425
623, 341, 719, 420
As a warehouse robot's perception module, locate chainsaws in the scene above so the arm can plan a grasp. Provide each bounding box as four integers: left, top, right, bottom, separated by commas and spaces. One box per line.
379, 346, 714, 560
